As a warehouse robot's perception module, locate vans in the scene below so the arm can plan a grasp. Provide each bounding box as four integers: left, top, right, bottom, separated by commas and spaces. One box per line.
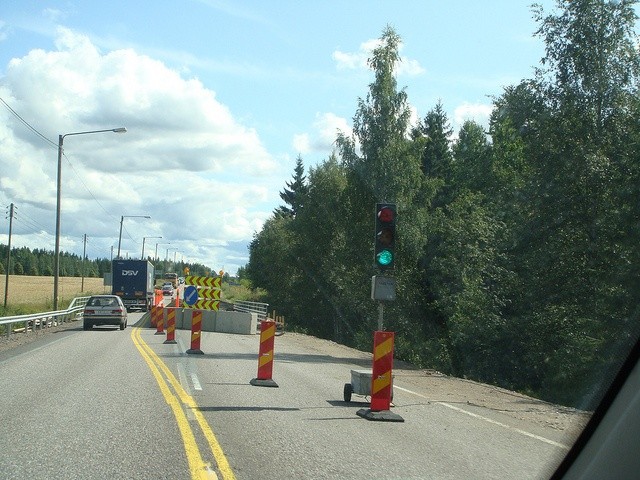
162, 282, 173, 288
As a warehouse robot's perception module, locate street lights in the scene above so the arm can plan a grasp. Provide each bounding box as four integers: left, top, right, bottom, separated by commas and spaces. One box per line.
54, 127, 127, 322
118, 216, 150, 260
142, 237, 162, 260
155, 243, 170, 260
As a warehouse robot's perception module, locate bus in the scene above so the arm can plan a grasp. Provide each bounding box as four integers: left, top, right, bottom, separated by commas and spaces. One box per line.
163, 273, 178, 289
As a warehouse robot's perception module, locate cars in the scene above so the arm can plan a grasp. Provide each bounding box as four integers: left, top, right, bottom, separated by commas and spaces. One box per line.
162, 287, 174, 296
84, 295, 127, 330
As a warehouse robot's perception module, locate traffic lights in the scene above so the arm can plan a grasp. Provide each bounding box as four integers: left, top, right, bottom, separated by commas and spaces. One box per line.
376, 204, 396, 267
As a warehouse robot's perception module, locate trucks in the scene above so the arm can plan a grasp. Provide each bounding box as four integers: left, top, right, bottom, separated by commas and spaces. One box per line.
112, 260, 154, 313
